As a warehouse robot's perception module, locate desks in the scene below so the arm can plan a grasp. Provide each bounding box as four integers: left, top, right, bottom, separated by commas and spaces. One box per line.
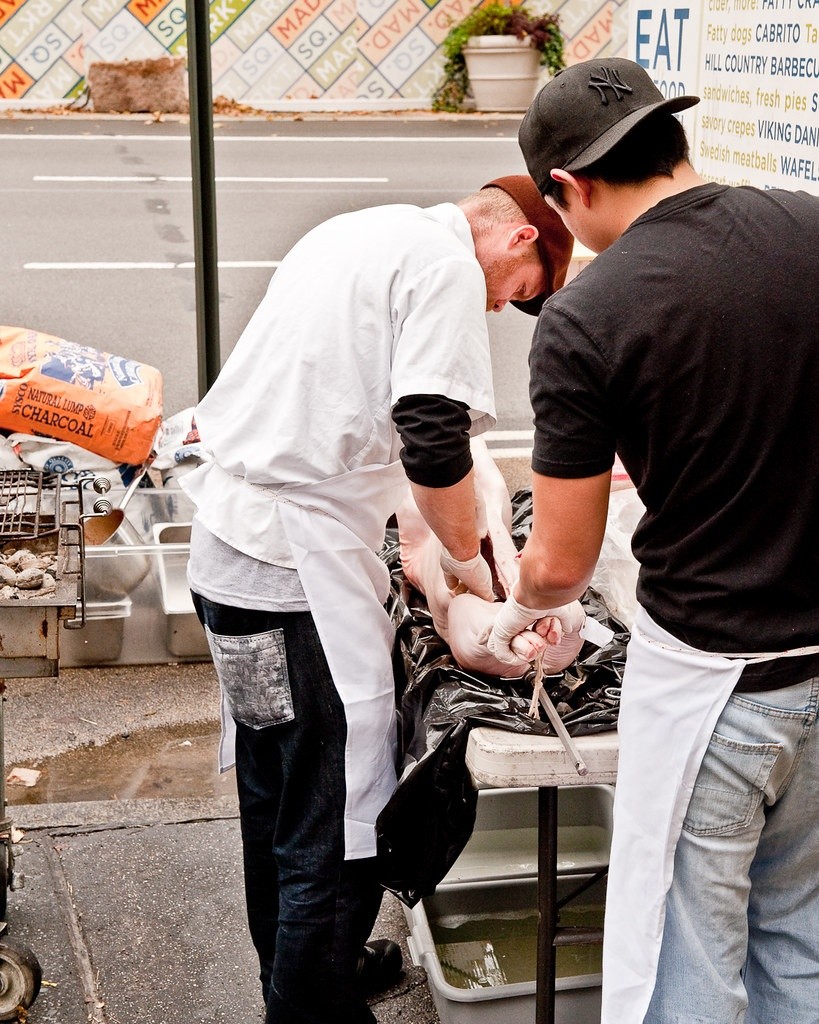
465, 726, 619, 1024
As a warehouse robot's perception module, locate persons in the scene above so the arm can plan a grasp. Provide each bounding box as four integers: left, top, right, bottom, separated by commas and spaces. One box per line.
487, 56, 819, 1024
177, 176, 575, 1024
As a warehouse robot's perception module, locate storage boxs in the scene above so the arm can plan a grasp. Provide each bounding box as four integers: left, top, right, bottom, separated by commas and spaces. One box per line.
56, 487, 212, 667
400, 783, 616, 1024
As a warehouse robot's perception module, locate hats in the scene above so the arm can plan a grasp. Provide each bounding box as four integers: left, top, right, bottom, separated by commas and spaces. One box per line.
518, 57, 699, 185
480, 175, 574, 316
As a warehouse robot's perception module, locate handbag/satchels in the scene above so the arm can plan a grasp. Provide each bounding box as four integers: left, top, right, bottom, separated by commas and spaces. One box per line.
0, 324, 165, 465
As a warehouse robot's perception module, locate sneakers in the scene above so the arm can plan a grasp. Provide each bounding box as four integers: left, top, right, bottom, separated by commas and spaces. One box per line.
351, 936, 404, 985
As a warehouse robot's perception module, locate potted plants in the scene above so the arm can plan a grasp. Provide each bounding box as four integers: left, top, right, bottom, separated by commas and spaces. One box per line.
430, 3, 567, 113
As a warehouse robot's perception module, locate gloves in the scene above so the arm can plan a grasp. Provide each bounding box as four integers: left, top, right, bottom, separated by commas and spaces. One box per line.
440, 542, 494, 603
478, 579, 573, 666
